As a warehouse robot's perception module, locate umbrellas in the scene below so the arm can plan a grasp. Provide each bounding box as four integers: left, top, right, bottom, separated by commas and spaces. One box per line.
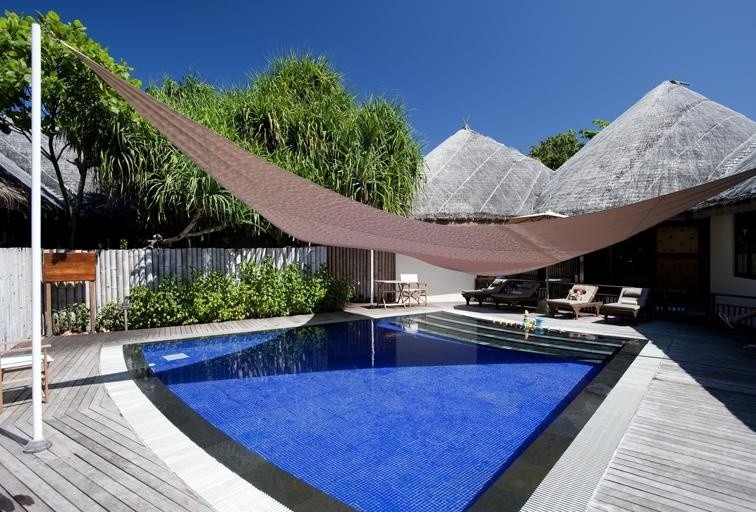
501, 208, 572, 300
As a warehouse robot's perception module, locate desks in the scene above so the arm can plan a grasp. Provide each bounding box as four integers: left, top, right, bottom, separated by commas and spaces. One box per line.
374, 280, 408, 310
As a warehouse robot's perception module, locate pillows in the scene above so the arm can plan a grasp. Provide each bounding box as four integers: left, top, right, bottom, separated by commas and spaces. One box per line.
623, 288, 643, 297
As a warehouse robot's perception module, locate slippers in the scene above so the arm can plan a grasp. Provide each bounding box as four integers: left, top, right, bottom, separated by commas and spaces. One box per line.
718, 311, 734, 328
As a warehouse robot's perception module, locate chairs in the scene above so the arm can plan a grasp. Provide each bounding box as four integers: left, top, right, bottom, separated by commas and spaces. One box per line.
603, 287, 649, 326
546, 284, 603, 320
0, 338, 54, 413
461, 279, 512, 307
492, 282, 544, 312
397, 274, 427, 308
